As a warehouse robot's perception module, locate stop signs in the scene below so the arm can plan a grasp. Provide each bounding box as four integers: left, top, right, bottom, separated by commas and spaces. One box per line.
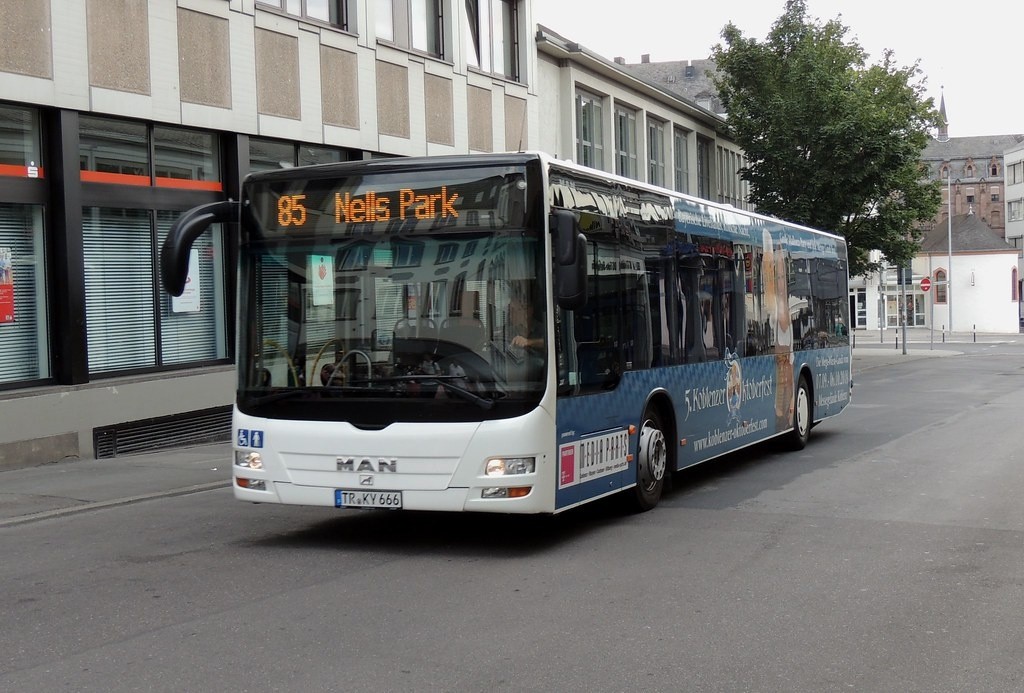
920, 279, 931, 291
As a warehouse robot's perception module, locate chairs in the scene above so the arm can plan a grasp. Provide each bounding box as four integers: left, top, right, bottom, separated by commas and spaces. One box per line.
387, 315, 486, 376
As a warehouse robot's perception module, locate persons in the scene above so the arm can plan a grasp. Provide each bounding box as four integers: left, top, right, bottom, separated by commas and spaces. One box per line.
505, 295, 545, 350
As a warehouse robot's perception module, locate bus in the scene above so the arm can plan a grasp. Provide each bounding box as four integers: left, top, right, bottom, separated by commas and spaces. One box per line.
160, 151, 850, 517
